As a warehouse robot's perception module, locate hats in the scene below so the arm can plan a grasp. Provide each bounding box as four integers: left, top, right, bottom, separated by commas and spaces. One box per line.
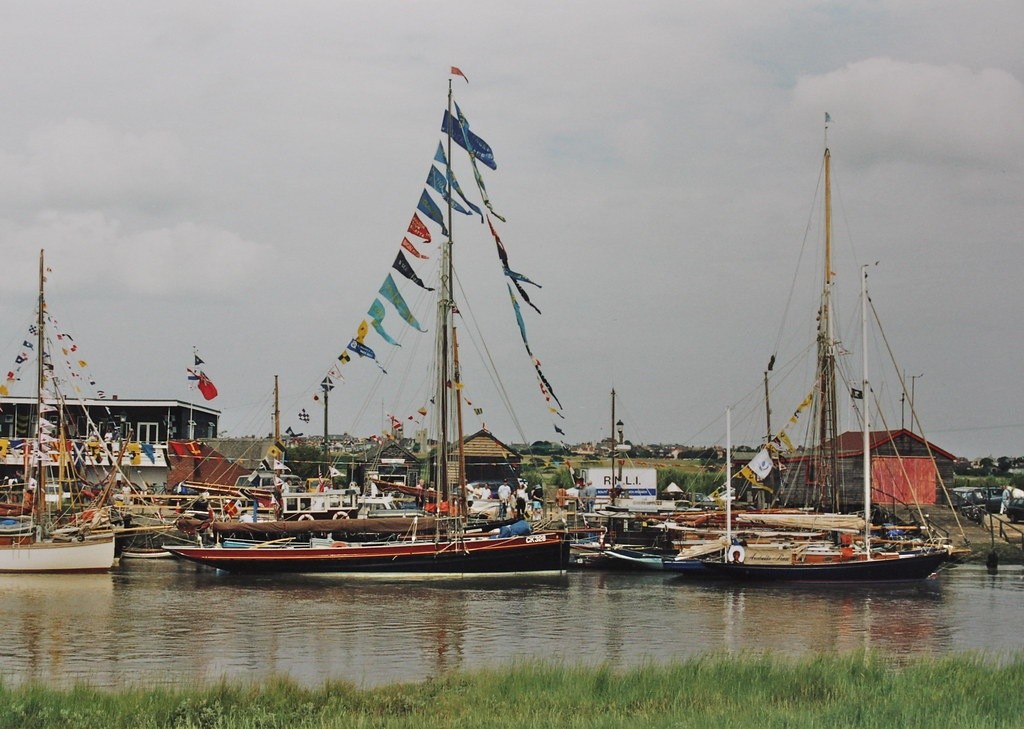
503, 478, 508, 482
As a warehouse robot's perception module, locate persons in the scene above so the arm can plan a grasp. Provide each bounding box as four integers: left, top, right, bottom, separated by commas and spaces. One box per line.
557, 483, 567, 510
579, 482, 596, 512
281, 478, 290, 493
17, 472, 36, 494
296, 482, 307, 493
121, 483, 131, 494
417, 480, 437, 507
325, 481, 332, 492
466, 479, 493, 508
105, 429, 112, 442
498, 479, 544, 519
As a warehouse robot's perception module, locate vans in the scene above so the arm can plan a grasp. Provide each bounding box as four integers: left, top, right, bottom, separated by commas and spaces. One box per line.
234, 474, 302, 493
357, 500, 435, 518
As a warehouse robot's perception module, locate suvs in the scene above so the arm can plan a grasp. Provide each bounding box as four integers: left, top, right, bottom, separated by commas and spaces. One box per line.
949, 487, 1024, 522
44, 484, 71, 502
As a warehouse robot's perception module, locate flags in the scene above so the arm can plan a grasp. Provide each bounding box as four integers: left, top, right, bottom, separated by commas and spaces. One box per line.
748, 381, 825, 479
338, 351, 351, 365
0, 299, 106, 399
0, 439, 201, 469
187, 355, 217, 400
418, 407, 428, 416
321, 377, 336, 391
298, 408, 312, 424
474, 408, 482, 415
224, 440, 284, 517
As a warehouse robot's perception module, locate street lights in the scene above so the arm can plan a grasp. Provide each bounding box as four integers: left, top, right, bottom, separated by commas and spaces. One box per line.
616, 420, 625, 481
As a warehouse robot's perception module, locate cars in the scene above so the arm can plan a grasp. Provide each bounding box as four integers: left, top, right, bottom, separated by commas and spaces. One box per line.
675, 493, 719, 510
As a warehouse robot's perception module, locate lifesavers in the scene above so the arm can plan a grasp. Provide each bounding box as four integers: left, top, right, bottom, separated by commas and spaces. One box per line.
727, 545, 745, 562
332, 511, 350, 520
297, 514, 314, 521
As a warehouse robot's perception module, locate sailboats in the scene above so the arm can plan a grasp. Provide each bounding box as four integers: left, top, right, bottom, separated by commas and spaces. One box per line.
662, 111, 973, 582
162, 67, 570, 578
0, 248, 115, 570
